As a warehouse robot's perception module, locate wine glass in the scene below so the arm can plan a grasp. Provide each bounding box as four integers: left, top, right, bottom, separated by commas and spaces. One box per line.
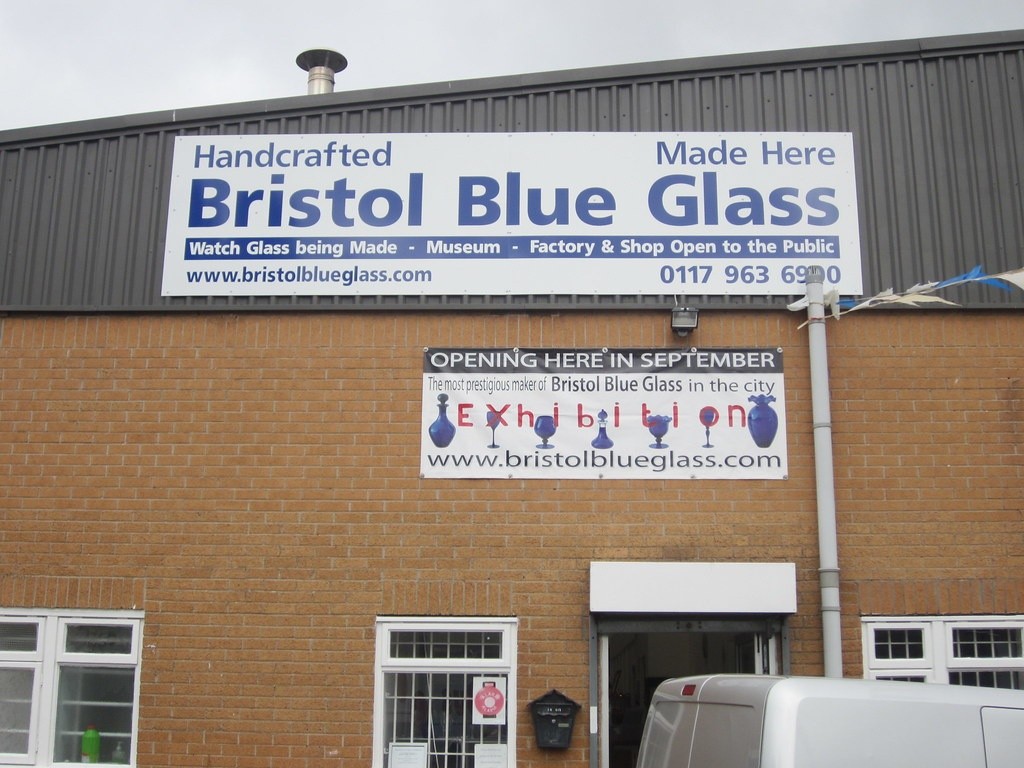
699, 410, 722, 454
488, 411, 502, 449
534, 416, 558, 449
645, 415, 670, 449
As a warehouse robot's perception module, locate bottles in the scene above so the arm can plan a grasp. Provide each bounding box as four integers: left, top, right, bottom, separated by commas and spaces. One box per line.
429, 394, 456, 447
81, 724, 100, 763
592, 410, 616, 449
748, 394, 778, 447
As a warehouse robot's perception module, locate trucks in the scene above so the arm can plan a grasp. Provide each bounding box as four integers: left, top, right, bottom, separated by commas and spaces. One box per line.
635, 674, 1024, 768
